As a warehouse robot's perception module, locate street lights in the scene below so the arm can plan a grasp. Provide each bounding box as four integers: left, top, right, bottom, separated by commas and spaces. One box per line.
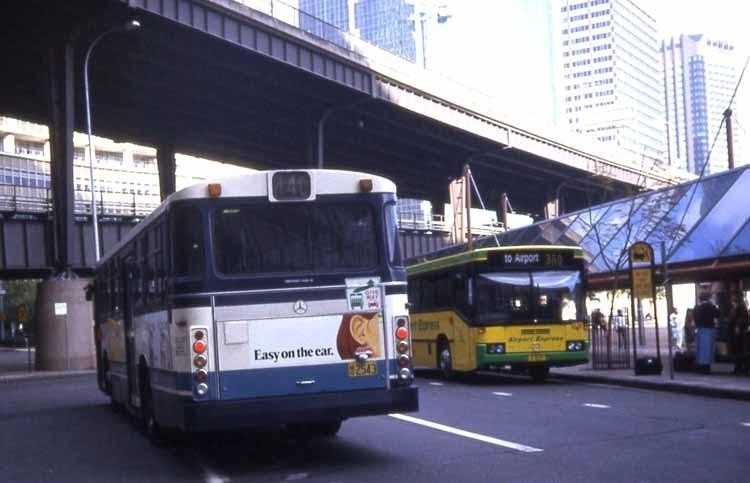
83, 19, 143, 265
0, 289, 8, 341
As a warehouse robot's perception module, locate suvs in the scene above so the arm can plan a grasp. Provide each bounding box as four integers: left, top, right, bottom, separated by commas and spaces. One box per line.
479, 220, 510, 240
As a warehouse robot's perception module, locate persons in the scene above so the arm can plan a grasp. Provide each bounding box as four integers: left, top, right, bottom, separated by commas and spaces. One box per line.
669, 308, 682, 350
727, 296, 750, 377
613, 309, 628, 350
693, 292, 720, 375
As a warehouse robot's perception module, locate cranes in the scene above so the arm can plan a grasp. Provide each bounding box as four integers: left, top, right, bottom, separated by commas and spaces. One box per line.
406, 0, 455, 72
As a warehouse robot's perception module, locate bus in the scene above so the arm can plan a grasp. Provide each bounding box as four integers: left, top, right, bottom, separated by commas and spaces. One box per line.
84, 168, 419, 448
405, 245, 587, 385
